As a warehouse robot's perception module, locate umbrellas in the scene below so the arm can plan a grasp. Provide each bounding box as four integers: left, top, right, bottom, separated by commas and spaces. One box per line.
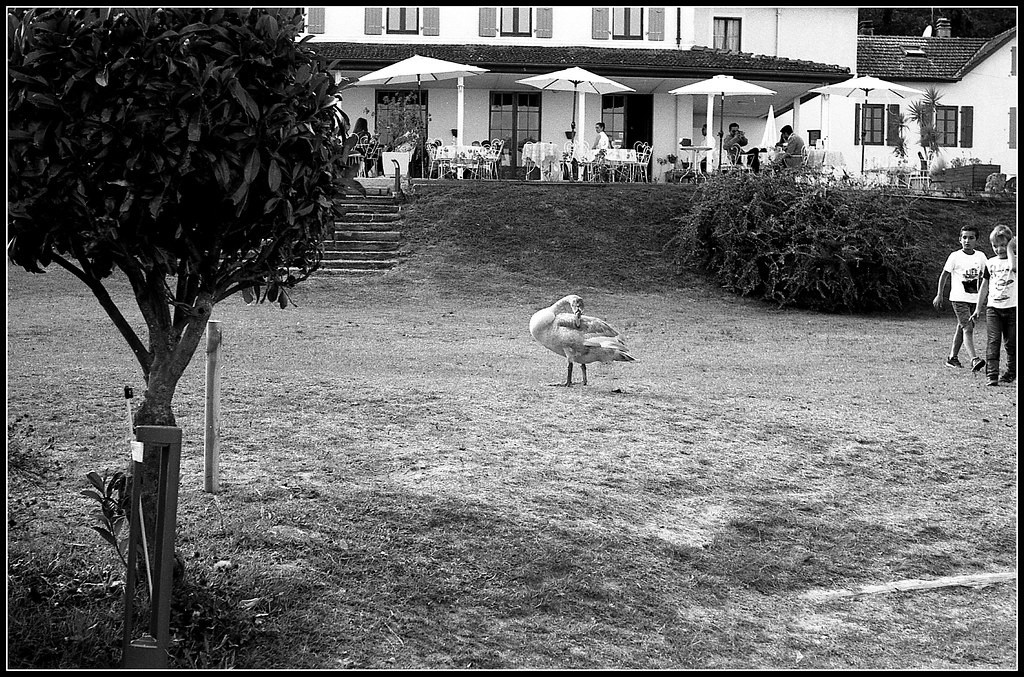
759, 104, 778, 164
349, 52, 491, 178
668, 75, 778, 169
808, 74, 928, 176
515, 66, 636, 161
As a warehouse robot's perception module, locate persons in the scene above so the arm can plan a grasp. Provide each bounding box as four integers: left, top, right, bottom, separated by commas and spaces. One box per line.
591, 122, 612, 149
378, 115, 419, 178
352, 116, 385, 178
693, 123, 807, 181
933, 223, 1016, 386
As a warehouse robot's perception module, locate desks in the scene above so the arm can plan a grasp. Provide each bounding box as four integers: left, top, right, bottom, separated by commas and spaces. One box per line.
524, 142, 559, 182
679, 146, 712, 185
436, 145, 488, 180
741, 153, 779, 175
587, 149, 637, 183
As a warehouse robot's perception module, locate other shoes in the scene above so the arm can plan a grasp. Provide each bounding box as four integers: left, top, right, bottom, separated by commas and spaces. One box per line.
999, 371, 1014, 382
987, 376, 998, 386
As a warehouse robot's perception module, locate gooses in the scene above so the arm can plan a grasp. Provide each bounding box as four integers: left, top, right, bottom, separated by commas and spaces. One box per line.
529, 294, 635, 387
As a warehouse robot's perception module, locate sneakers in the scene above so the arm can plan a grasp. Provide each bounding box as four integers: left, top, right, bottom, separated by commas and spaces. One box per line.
970, 356, 986, 372
946, 356, 963, 369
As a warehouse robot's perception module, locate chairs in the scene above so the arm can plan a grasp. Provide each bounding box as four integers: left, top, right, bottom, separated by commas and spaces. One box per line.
425, 138, 505, 180
721, 141, 825, 173
347, 133, 385, 178
559, 141, 654, 184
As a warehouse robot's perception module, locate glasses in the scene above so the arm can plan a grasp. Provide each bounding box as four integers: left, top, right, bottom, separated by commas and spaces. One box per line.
731, 130, 738, 133
